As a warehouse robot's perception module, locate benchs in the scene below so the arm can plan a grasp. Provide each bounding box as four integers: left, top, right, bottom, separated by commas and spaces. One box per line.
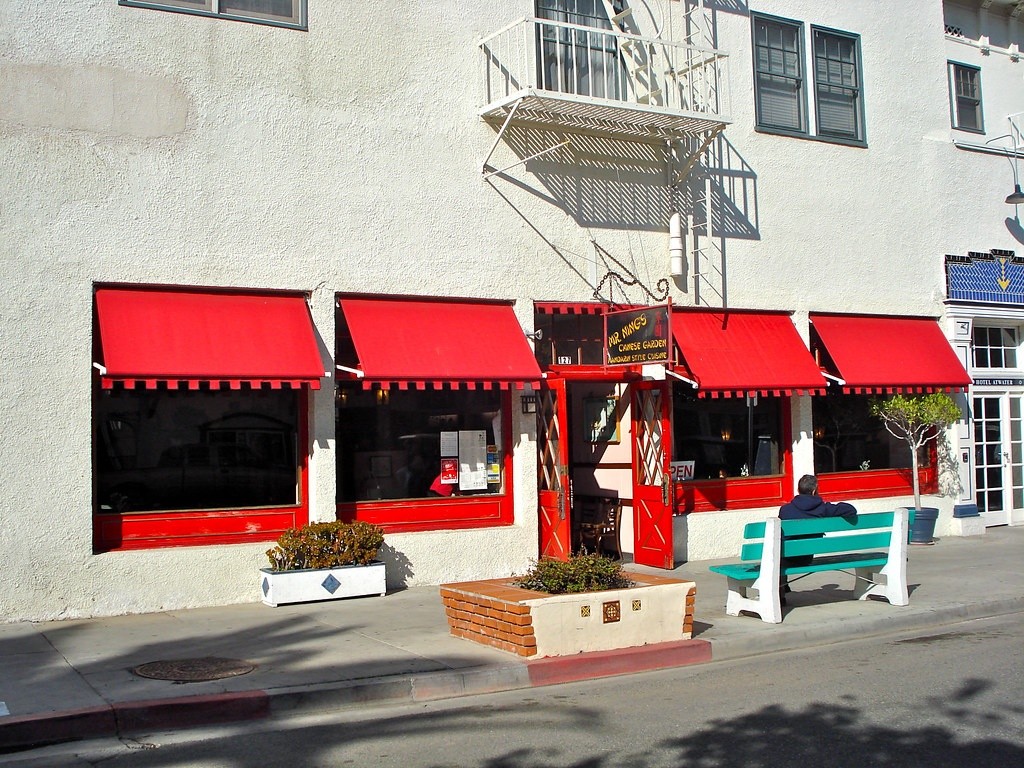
710, 508, 916, 623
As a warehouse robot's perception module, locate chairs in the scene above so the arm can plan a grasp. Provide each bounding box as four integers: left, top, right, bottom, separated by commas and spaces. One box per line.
572, 500, 622, 559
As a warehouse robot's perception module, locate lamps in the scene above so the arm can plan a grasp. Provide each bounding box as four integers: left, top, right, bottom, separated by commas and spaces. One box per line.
985, 134, 1024, 204
521, 394, 537, 413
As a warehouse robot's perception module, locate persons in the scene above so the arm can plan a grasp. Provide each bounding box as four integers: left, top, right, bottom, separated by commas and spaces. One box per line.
778, 474, 857, 593
426, 475, 454, 498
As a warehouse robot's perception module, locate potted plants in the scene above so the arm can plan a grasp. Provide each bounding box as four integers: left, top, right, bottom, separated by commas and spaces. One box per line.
440, 547, 695, 658
260, 519, 386, 606
871, 391, 963, 543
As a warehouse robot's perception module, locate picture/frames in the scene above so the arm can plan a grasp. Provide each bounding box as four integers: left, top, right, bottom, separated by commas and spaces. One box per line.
582, 395, 621, 443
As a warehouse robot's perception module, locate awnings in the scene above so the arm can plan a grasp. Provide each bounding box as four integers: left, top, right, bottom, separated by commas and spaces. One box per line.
91, 287, 332, 391
336, 297, 543, 392
669, 310, 828, 398
810, 310, 973, 396
